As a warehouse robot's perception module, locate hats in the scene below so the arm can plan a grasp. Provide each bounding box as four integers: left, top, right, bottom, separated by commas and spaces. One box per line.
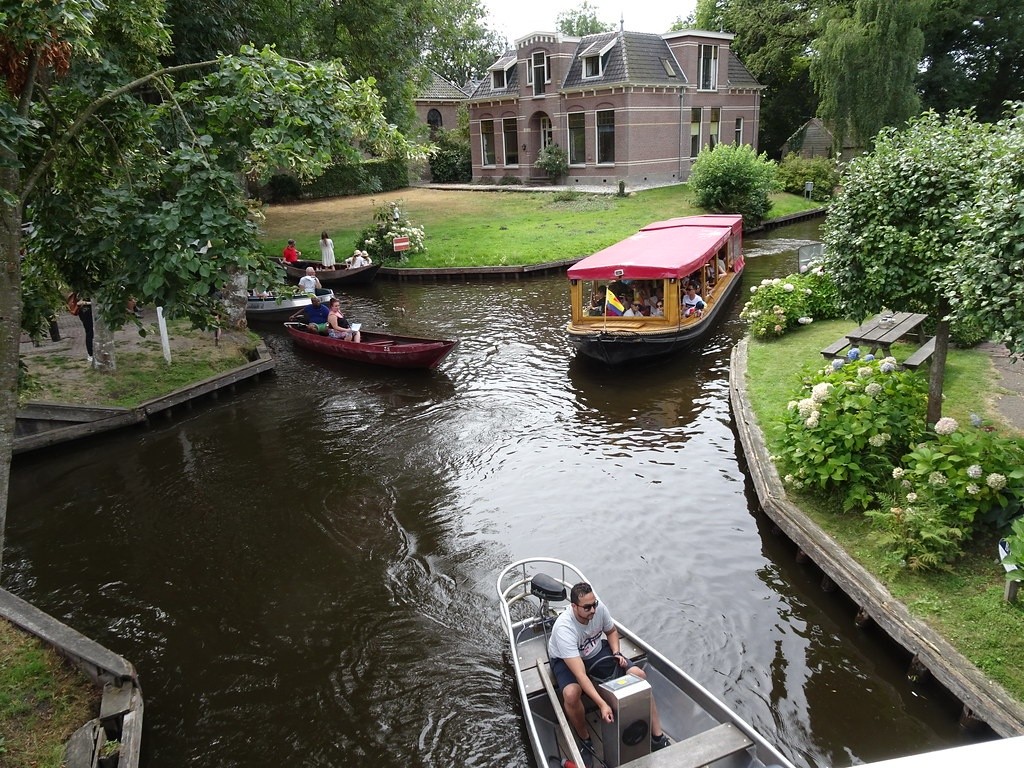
361, 251, 368, 256
354, 250, 361, 257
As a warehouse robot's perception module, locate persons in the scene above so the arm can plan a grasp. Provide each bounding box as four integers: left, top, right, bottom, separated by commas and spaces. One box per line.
283, 239, 301, 267
328, 298, 360, 344
705, 249, 727, 289
589, 280, 664, 317
298, 266, 321, 295
289, 296, 329, 335
252, 287, 272, 296
78, 297, 94, 361
361, 250, 372, 264
320, 231, 335, 270
345, 250, 364, 271
548, 582, 671, 768
680, 270, 707, 319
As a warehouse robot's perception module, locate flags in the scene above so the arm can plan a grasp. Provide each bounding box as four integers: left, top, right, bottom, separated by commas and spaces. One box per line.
607, 289, 624, 315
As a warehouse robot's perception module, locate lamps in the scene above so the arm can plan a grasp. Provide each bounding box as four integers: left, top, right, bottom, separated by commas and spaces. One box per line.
522, 144, 526, 150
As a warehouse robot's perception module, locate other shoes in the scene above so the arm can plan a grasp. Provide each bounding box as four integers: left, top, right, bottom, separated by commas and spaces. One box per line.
87, 355, 92, 361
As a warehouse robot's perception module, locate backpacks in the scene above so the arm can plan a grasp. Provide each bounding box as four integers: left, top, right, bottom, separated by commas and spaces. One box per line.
67, 292, 80, 315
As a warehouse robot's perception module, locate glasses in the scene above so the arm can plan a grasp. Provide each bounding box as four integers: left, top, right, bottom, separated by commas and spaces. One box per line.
571, 599, 598, 611
632, 304, 641, 307
656, 305, 663, 308
687, 288, 696, 291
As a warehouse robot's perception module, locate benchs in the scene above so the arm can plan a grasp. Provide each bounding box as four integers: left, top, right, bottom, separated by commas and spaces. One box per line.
615, 722, 754, 768
903, 334, 952, 367
520, 636, 647, 698
820, 337, 850, 356
360, 339, 394, 346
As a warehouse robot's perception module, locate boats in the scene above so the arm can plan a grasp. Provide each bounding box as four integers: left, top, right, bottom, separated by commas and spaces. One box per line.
246, 286, 336, 326
497, 556, 796, 768
266, 256, 384, 289
564, 213, 746, 368
282, 318, 463, 373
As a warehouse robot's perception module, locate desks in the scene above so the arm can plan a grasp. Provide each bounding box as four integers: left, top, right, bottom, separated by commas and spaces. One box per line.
824, 309, 934, 375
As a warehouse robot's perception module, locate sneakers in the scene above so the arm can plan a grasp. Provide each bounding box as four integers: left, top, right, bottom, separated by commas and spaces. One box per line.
578, 738, 594, 768
651, 735, 671, 751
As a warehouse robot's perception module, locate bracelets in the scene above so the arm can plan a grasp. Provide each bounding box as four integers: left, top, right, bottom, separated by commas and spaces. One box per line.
614, 652, 622, 656
